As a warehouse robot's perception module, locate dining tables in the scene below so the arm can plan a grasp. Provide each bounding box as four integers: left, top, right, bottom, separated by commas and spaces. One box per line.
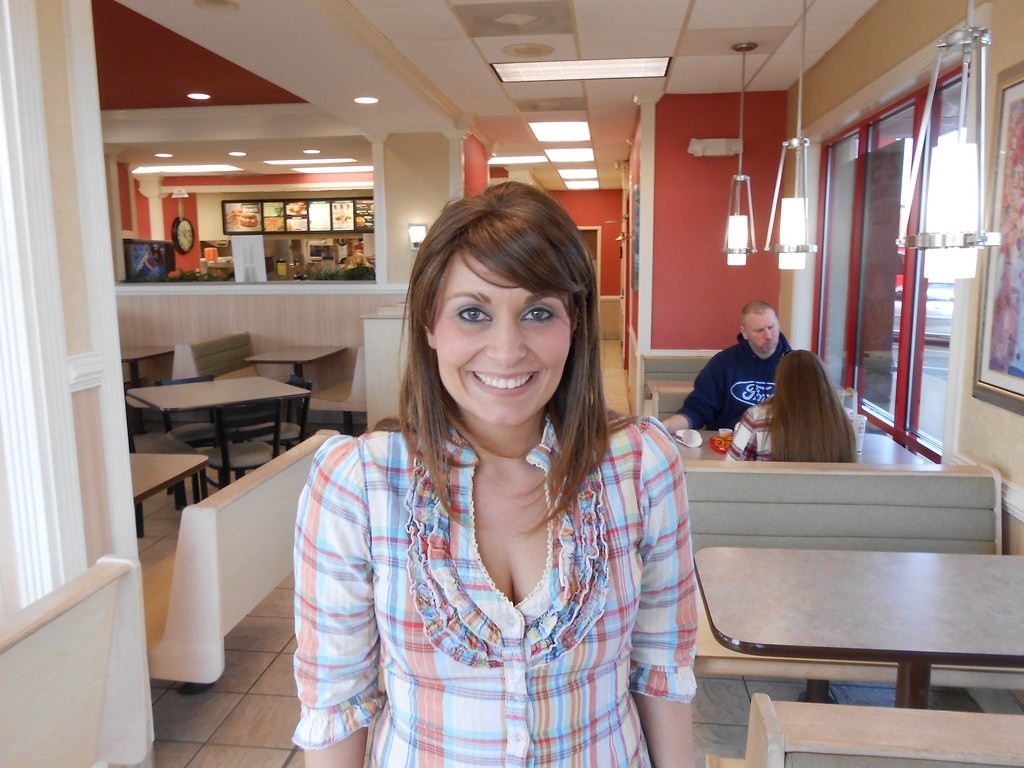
662, 430, 927, 467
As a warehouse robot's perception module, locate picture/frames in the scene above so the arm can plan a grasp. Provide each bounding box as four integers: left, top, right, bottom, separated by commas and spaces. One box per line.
971, 61, 1024, 416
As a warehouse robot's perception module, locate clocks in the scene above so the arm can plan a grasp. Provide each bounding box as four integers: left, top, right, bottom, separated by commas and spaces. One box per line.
172, 216, 195, 254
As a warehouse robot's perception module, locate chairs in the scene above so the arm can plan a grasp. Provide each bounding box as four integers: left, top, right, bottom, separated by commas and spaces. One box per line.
152, 374, 312, 510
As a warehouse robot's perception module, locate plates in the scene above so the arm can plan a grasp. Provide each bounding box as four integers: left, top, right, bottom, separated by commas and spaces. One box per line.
709, 436, 733, 454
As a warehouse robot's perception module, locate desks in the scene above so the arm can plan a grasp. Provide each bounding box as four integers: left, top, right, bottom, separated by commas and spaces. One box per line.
129, 453, 210, 538
695, 546, 1024, 710
119, 344, 174, 435
245, 344, 348, 436
127, 377, 312, 510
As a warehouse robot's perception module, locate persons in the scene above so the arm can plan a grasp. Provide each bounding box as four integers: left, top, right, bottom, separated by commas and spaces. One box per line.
726, 349, 860, 463
341, 254, 375, 279
290, 181, 698, 768
662, 299, 794, 434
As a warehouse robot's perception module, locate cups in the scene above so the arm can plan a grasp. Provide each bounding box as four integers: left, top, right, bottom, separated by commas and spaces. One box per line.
849, 414, 867, 454
719, 429, 733, 436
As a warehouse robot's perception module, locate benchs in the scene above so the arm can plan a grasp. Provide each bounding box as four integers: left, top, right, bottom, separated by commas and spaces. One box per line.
634, 348, 1023, 768
303, 343, 366, 436
169, 330, 264, 424
142, 430, 342, 696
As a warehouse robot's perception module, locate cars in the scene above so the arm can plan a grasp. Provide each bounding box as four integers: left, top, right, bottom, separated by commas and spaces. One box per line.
893, 275, 956, 343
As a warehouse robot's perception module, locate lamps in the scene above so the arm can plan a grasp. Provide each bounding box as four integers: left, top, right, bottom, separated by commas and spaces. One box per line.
896, 0, 1000, 283
409, 226, 426, 251
764, 0, 818, 272
721, 42, 758, 266
